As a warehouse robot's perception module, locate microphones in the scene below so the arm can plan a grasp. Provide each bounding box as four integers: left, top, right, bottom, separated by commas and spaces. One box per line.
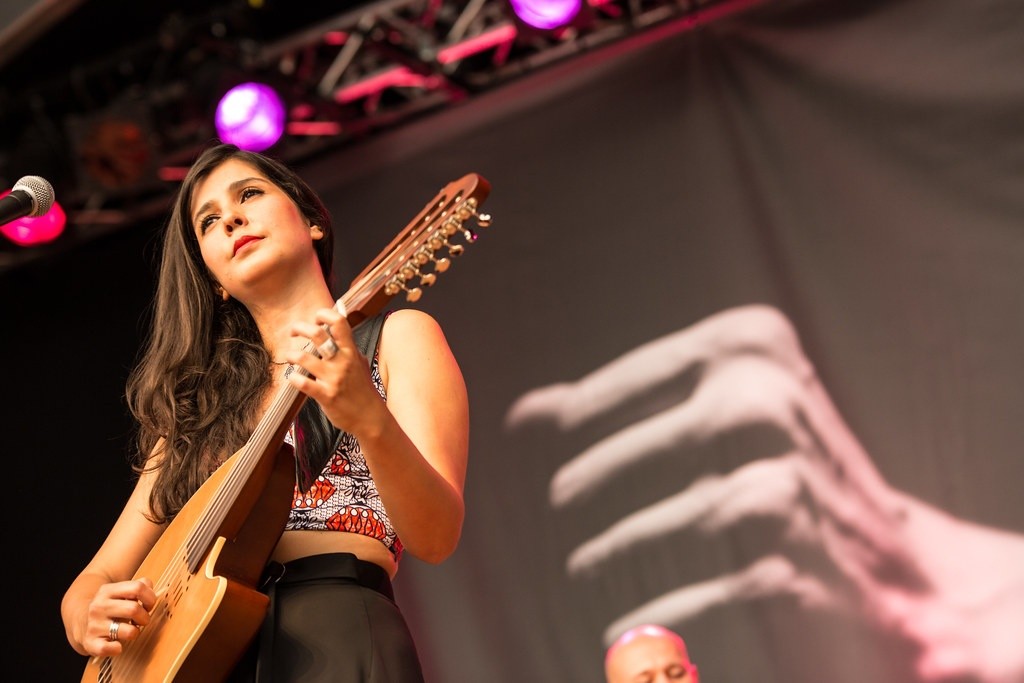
0, 175, 55, 226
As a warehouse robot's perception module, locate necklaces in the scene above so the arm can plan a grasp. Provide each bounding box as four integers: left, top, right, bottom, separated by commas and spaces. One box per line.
270, 339, 314, 379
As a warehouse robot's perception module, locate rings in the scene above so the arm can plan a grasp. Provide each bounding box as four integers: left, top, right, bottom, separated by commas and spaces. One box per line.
318, 337, 339, 360
110, 619, 123, 640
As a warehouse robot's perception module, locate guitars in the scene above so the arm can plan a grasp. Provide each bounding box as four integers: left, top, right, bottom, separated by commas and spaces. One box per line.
75, 168, 498, 683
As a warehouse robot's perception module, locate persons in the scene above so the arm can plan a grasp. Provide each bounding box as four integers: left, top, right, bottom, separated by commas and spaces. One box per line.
605, 624, 700, 683
503, 303, 1024, 683
61, 143, 470, 683
